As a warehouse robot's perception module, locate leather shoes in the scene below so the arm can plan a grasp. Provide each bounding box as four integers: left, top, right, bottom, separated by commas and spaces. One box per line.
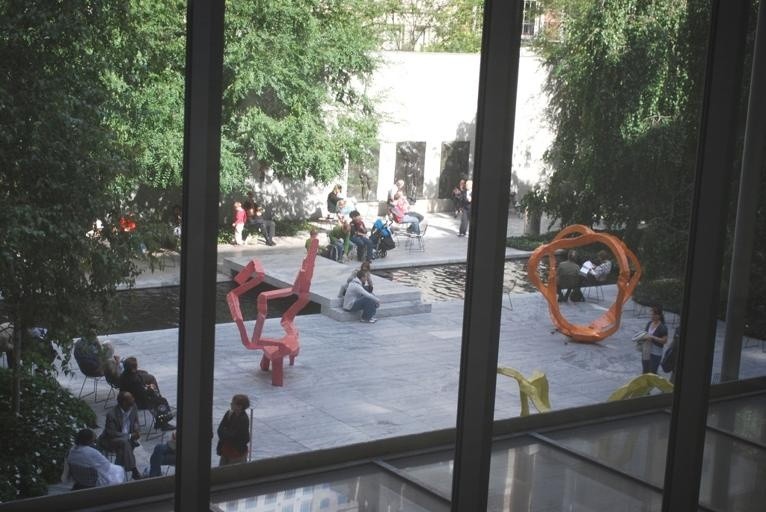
131, 473, 144, 480
154, 413, 177, 430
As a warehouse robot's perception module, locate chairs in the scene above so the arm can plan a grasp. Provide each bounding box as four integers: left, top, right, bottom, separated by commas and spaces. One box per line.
503, 280, 516, 311
69, 356, 178, 491
405, 223, 427, 252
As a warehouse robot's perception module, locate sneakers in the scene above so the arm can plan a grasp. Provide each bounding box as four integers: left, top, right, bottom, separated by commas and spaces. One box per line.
405, 230, 422, 238
360, 318, 377, 322
557, 292, 567, 302
266, 240, 275, 246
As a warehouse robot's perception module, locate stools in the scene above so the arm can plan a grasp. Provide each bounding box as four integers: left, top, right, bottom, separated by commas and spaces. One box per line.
318, 217, 334, 234
583, 281, 606, 302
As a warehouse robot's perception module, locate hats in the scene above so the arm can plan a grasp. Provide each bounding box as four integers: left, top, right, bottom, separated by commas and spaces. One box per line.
649, 304, 663, 313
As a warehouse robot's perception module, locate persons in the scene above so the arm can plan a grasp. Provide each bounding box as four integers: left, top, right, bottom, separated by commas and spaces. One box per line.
636, 305, 668, 394
216, 394, 251, 467
228, 200, 277, 255
583, 250, 613, 286
452, 178, 473, 236
555, 249, 583, 302
510, 180, 522, 210
661, 327, 680, 385
85, 203, 182, 250
26, 329, 178, 494
304, 172, 424, 324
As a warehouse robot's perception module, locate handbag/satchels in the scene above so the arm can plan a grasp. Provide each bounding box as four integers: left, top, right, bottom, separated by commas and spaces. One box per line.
661, 348, 673, 372
383, 237, 395, 250
570, 290, 584, 301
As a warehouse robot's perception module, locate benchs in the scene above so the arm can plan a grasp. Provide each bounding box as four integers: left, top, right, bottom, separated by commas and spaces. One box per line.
632, 296, 680, 329
